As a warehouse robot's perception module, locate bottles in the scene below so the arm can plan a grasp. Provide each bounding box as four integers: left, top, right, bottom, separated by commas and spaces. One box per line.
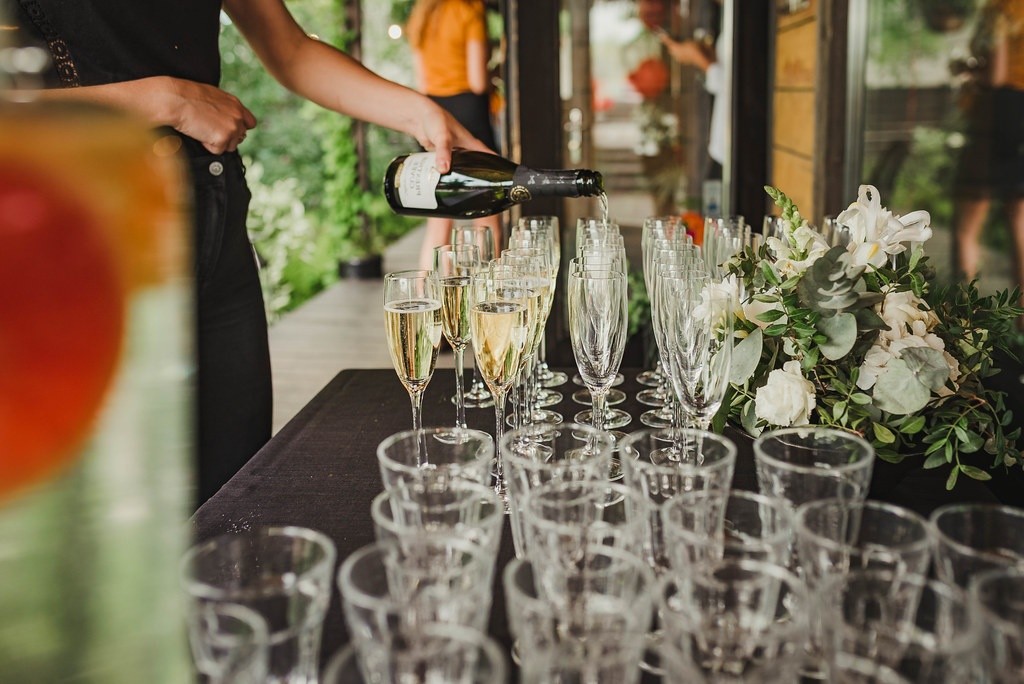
383, 148, 602, 221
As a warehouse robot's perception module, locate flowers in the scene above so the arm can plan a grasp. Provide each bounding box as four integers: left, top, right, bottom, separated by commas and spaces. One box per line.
694, 186, 1024, 490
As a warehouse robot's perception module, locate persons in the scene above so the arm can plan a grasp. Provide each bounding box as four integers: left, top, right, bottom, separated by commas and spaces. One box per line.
1, 0, 499, 518
409, 0, 503, 295
954, 0, 1024, 290
658, 0, 724, 218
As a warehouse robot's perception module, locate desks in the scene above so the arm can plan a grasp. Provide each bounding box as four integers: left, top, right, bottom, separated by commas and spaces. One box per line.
188, 368, 1024, 684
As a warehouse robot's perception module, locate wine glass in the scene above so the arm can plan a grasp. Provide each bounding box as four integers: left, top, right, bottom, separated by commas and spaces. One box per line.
182, 215, 1024, 684
